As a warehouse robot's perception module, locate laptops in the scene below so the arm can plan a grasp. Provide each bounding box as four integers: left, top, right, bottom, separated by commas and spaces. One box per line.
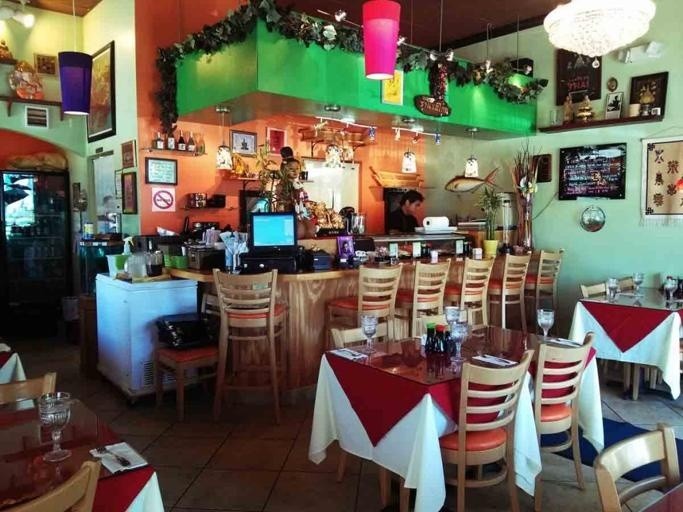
244, 212, 298, 260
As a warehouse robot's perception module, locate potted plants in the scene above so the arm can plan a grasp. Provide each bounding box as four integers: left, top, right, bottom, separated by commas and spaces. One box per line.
473, 187, 507, 257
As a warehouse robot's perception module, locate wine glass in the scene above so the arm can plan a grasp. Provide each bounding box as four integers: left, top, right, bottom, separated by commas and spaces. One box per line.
632, 272, 645, 294
360, 313, 378, 355
536, 308, 556, 344
449, 321, 467, 365
632, 295, 642, 308
663, 279, 678, 304
37, 391, 74, 464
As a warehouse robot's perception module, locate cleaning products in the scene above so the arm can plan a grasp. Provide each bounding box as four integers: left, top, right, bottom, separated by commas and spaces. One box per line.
121, 237, 135, 256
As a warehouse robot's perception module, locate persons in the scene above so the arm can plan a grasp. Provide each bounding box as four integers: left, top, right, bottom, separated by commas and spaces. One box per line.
274, 146, 319, 226
562, 94, 573, 121
385, 190, 423, 236
576, 94, 593, 108
339, 241, 351, 258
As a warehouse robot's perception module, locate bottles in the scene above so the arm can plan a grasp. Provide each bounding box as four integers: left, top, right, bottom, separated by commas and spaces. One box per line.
501, 242, 512, 255
424, 322, 455, 354
7, 176, 62, 277
154, 127, 207, 153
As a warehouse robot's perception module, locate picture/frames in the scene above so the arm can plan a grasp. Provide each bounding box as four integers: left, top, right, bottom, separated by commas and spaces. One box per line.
34, 53, 57, 77
122, 141, 137, 168
85, 40, 116, 144
122, 172, 138, 215
382, 69, 404, 107
555, 48, 669, 201
145, 157, 179, 186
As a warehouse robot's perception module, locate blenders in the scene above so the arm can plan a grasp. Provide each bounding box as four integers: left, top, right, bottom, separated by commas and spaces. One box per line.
347, 210, 375, 254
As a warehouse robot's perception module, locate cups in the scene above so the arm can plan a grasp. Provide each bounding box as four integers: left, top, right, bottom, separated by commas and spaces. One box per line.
605, 277, 619, 302
548, 109, 560, 127
629, 103, 661, 117
443, 304, 460, 322
126, 251, 163, 280
173, 255, 189, 270
299, 171, 308, 180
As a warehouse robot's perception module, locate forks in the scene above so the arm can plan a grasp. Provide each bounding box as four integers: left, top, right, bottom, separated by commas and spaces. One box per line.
476, 351, 510, 363
93, 442, 131, 468
336, 347, 361, 358
550, 333, 581, 346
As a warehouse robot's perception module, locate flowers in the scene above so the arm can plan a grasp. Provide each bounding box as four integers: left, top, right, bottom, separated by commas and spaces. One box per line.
513, 138, 543, 231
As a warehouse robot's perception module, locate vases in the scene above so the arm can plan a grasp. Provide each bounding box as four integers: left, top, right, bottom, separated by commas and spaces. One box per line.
517, 187, 535, 253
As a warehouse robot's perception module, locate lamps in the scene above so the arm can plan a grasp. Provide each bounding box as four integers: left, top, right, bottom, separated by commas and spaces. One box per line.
316, 0, 534, 80
59, 0, 93, 116
543, 0, 662, 67
215, 104, 480, 176
0, 0, 35, 29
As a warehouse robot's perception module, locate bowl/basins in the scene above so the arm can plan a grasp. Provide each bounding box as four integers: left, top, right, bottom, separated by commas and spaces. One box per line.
157, 311, 220, 350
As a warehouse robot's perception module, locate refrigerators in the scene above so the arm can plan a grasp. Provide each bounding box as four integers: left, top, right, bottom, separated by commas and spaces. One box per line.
92, 271, 201, 399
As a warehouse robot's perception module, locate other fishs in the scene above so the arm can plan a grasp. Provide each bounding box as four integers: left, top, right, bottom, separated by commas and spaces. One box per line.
444, 166, 504, 194
